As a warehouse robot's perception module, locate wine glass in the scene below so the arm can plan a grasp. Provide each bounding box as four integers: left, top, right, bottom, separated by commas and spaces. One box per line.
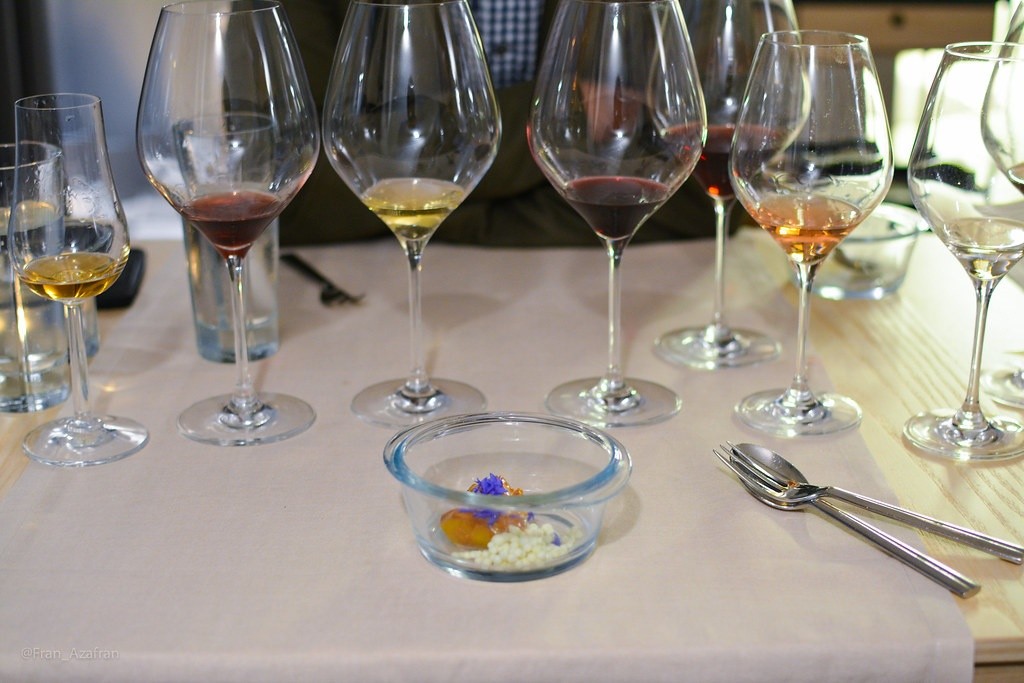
257, 344, 317, 444
905, 40, 1024, 460
645, 1, 811, 371
526, 0, 709, 430
321, 0, 502, 434
13, 92, 151, 466
735, 24, 895, 436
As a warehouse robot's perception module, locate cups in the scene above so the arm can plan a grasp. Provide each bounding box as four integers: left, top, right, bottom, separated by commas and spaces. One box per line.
0, 142, 72, 413
183, 211, 283, 362
978, 0, 1023, 195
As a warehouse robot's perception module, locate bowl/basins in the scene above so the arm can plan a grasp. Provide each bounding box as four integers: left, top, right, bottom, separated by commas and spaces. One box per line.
384, 414, 635, 583
788, 212, 918, 299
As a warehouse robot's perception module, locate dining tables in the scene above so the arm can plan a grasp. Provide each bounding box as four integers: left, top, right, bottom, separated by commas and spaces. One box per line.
1, 236, 1024, 683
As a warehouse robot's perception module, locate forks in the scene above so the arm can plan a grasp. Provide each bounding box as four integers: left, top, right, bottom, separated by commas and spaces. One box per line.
711, 439, 1024, 564
280, 252, 364, 306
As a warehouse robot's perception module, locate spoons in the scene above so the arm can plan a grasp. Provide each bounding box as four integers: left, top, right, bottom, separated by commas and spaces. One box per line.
732, 443, 980, 599
